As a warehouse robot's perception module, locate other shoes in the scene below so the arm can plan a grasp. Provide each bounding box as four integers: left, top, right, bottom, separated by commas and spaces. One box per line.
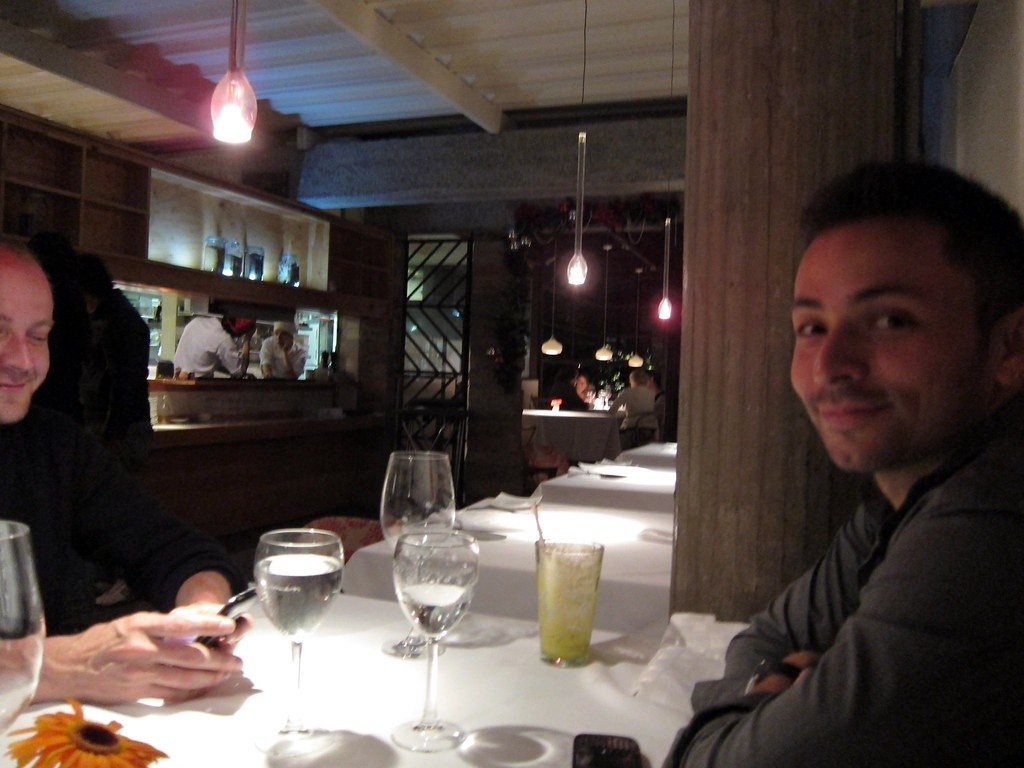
96, 580, 136, 606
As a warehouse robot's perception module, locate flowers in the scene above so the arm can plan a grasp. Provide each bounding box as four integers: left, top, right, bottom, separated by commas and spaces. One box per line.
5, 698, 168, 768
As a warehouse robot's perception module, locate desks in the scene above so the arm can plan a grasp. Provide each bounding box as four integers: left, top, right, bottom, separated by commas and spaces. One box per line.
520, 408, 626, 474
0, 439, 759, 768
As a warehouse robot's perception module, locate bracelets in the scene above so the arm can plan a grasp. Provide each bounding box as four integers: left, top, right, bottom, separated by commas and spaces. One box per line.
752, 658, 803, 682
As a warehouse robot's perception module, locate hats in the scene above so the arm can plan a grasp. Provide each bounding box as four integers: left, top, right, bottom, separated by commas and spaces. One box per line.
273, 320, 294, 338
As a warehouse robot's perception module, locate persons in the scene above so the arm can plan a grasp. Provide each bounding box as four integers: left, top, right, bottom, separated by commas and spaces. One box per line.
664, 154, 1024, 768
1, 229, 249, 704
607, 370, 674, 444
548, 366, 611, 413
259, 319, 310, 379
173, 305, 250, 377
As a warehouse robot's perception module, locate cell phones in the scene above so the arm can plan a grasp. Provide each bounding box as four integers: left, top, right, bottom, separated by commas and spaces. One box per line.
193, 587, 257, 648
572, 733, 642, 768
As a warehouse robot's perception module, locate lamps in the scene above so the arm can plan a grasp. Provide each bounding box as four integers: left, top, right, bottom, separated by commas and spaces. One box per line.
628, 268, 643, 367
568, 0, 587, 286
211, 0, 257, 144
658, 213, 672, 319
541, 235, 562, 354
595, 243, 613, 361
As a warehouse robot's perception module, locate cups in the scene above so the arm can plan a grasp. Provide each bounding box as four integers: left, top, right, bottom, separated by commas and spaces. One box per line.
599, 388, 613, 411
537, 543, 604, 669
0, 521, 47, 736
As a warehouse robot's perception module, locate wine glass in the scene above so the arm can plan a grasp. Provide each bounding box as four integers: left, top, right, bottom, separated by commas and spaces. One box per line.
393, 529, 480, 754
382, 451, 455, 660
254, 527, 344, 756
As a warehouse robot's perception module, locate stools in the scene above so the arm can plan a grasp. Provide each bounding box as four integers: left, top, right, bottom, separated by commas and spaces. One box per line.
522, 446, 569, 482
299, 515, 382, 562
